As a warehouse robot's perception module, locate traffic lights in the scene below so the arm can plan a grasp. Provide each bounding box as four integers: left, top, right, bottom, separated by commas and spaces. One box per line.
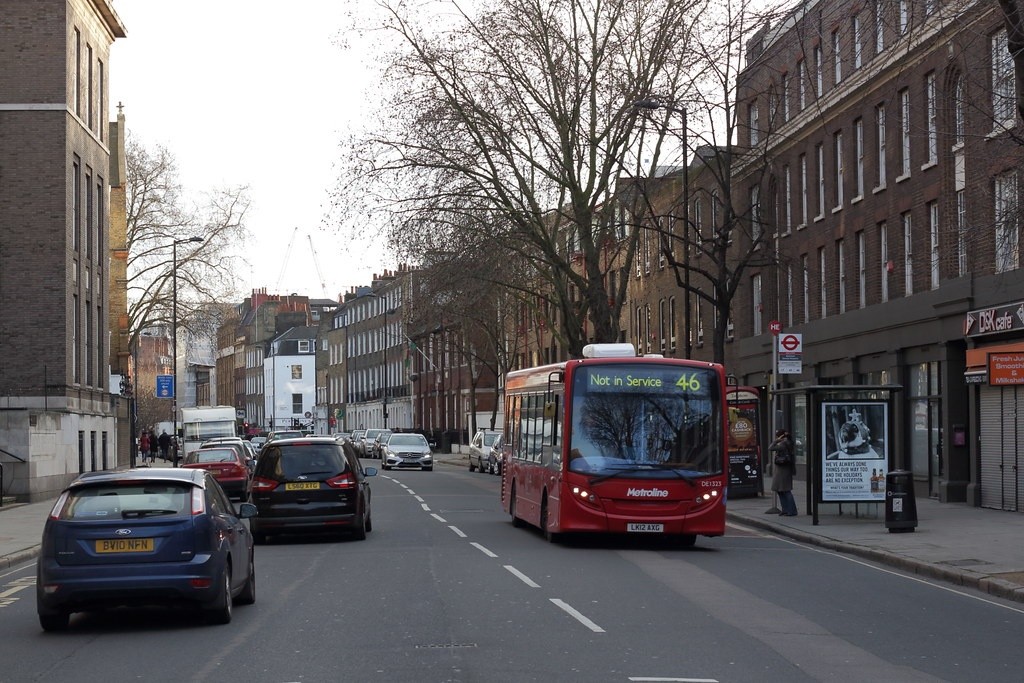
244, 423, 250, 433
269, 421, 272, 428
294, 418, 299, 430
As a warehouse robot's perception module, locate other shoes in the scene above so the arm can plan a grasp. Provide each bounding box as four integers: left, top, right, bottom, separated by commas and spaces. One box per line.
779, 512, 788, 516
785, 512, 798, 516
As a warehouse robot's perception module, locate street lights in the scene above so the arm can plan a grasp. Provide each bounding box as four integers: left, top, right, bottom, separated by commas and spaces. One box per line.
173, 236, 205, 467
633, 99, 690, 360
365, 290, 395, 427
135, 331, 152, 457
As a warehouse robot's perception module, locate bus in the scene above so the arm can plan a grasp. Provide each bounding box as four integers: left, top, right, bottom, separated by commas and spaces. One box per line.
499, 344, 741, 547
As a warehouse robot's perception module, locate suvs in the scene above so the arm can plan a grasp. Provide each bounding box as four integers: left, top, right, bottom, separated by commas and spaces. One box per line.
469, 431, 504, 474
251, 433, 377, 545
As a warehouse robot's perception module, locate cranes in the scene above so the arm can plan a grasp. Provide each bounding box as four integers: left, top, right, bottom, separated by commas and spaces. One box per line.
274, 227, 298, 296
307, 235, 328, 300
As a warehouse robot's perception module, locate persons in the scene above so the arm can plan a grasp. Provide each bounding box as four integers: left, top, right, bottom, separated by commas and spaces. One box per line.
766, 428, 798, 516
157, 428, 173, 463
138, 431, 150, 462
147, 429, 158, 462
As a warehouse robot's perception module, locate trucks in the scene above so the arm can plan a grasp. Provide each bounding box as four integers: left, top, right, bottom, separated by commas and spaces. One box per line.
178, 405, 238, 463
153, 421, 181, 441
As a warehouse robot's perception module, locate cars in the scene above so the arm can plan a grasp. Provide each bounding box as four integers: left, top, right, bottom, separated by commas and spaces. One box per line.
267, 427, 393, 459
487, 432, 505, 476
380, 433, 434, 472
180, 447, 252, 504
157, 435, 184, 462
37, 468, 259, 635
199, 436, 269, 477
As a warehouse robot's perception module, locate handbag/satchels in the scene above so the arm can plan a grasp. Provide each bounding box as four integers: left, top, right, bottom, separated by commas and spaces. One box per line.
775, 442, 794, 465
